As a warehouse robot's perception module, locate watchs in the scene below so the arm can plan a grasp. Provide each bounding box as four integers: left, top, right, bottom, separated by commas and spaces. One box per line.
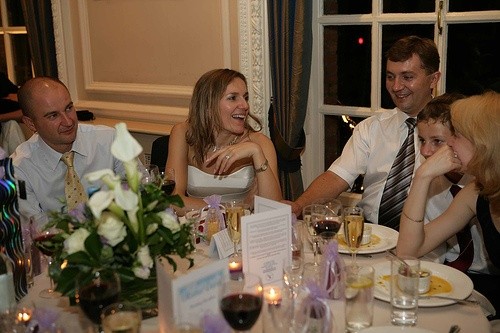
255, 160, 268, 173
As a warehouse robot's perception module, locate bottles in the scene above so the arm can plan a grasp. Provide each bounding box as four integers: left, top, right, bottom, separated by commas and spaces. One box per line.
320, 256, 346, 300
206, 209, 220, 239
301, 295, 332, 333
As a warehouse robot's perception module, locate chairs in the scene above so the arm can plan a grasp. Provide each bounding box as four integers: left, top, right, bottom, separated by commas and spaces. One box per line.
149, 137, 188, 196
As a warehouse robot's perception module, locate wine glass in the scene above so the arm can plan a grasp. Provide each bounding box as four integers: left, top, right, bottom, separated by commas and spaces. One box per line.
224, 199, 245, 259
342, 207, 366, 270
30, 212, 64, 299
303, 198, 363, 272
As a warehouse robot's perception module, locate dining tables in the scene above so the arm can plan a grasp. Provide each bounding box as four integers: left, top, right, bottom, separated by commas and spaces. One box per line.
18, 220, 500, 333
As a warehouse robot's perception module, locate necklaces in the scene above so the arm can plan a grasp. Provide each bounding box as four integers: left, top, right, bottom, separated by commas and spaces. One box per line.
209, 134, 242, 152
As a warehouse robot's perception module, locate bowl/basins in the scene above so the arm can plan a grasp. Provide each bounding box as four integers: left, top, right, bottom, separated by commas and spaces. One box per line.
361, 227, 372, 244
398, 269, 432, 294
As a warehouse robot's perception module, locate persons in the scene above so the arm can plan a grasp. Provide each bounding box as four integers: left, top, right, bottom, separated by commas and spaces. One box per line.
0, 69, 282, 231
280, 37, 500, 311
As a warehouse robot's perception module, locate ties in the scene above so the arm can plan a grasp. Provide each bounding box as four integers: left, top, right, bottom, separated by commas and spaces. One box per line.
378, 118, 416, 230
60, 151, 87, 211
444, 184, 474, 273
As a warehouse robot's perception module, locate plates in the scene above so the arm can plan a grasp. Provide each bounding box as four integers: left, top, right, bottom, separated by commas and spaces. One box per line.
358, 260, 474, 307
307, 221, 400, 252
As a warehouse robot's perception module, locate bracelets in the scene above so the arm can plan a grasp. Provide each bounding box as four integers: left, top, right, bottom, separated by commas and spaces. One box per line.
402, 209, 424, 222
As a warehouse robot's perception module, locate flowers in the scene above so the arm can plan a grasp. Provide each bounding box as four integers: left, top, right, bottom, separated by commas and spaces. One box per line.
34, 122, 200, 323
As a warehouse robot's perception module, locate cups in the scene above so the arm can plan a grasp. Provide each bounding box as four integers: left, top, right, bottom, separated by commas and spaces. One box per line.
390, 256, 420, 318
102, 303, 142, 332
343, 263, 375, 328
142, 164, 176, 195
217, 257, 295, 333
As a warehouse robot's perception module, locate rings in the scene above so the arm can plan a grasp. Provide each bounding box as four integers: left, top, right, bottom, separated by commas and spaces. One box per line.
225, 155, 229, 160
